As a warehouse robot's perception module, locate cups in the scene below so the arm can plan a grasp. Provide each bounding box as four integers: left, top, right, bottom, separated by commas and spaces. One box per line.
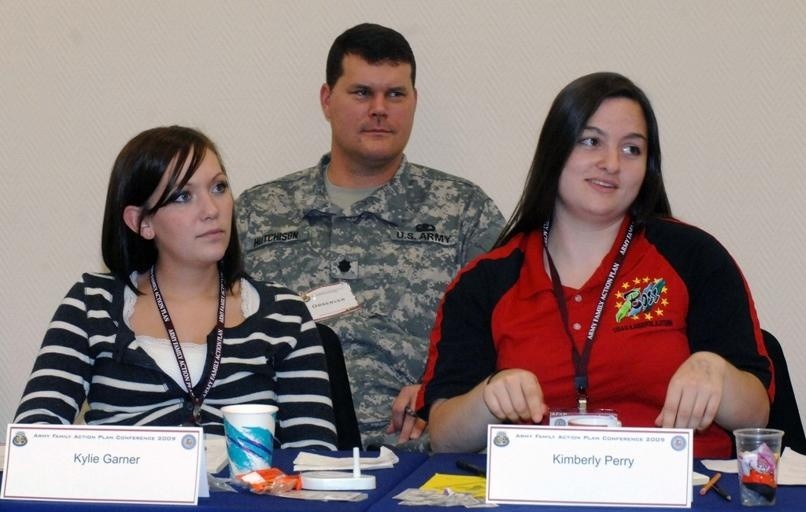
219, 404, 279, 481
732, 428, 784, 508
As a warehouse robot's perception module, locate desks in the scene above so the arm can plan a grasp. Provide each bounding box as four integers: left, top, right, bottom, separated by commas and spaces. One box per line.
1, 449, 806, 512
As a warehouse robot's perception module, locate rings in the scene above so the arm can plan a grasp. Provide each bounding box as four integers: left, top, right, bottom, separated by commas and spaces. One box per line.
405, 407, 417, 416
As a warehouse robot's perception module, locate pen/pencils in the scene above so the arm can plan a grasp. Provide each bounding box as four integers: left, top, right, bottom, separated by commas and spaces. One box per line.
699, 473, 732, 501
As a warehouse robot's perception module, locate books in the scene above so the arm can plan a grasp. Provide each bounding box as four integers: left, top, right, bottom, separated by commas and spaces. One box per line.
199, 433, 229, 474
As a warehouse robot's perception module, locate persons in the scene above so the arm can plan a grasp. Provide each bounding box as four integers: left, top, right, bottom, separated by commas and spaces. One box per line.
220, 20, 515, 458
414, 69, 780, 463
7, 125, 340, 479
